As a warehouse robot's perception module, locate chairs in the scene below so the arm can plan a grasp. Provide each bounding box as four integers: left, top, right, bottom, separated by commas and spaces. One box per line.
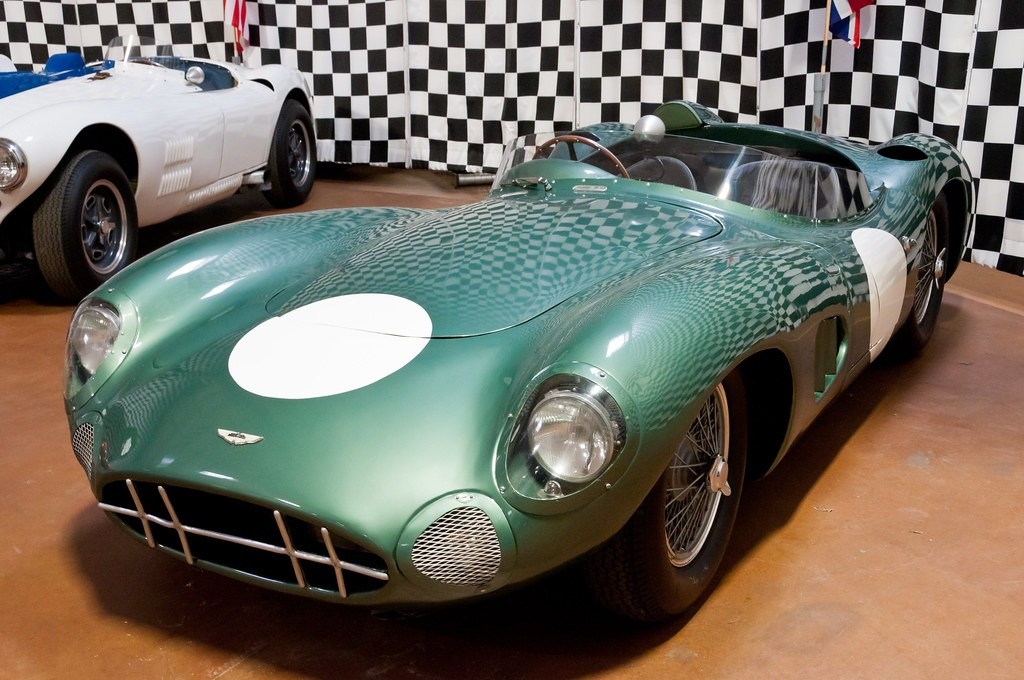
619, 156, 697, 190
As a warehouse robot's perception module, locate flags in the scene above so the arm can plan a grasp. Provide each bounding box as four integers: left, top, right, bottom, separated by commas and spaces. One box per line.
829, 0, 878, 50
225, 1, 254, 56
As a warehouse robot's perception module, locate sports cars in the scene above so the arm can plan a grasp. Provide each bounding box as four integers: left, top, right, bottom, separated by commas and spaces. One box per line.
62, 99, 980, 629
0, 36, 320, 301
